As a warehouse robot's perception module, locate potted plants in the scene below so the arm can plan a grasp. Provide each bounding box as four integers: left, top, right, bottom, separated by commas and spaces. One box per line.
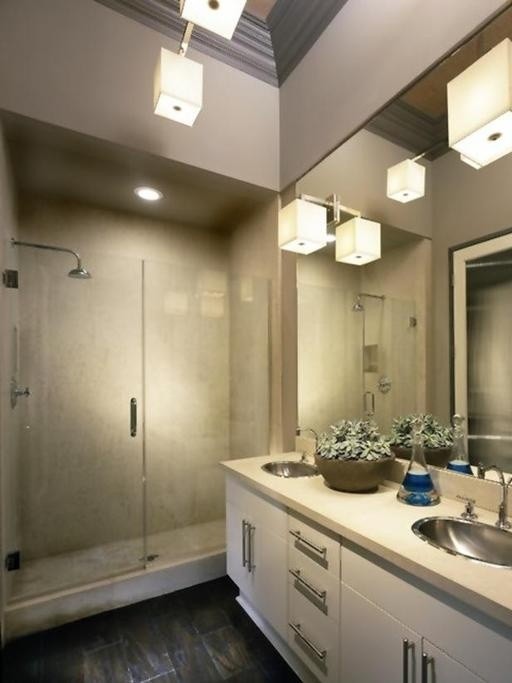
311, 419, 396, 492
389, 412, 455, 477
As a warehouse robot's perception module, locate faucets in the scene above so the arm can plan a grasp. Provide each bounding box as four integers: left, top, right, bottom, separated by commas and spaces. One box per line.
294, 426, 320, 469
477, 463, 507, 526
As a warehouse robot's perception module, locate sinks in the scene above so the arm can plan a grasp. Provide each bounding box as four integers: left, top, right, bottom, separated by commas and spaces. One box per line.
262, 459, 322, 481
412, 515, 512, 569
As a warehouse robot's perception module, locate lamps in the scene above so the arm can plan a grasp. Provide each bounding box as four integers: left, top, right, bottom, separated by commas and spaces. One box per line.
384, 157, 428, 206
151, 45, 207, 130
179, 1, 249, 42
447, 38, 512, 170
277, 198, 328, 256
335, 216, 382, 267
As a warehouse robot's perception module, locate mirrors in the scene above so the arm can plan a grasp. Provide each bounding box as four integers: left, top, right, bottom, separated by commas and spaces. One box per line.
292, 0, 512, 486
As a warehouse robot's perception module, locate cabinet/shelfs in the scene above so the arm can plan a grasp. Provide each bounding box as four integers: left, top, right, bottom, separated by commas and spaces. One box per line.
287, 512, 341, 682
217, 474, 286, 662
341, 538, 511, 683
